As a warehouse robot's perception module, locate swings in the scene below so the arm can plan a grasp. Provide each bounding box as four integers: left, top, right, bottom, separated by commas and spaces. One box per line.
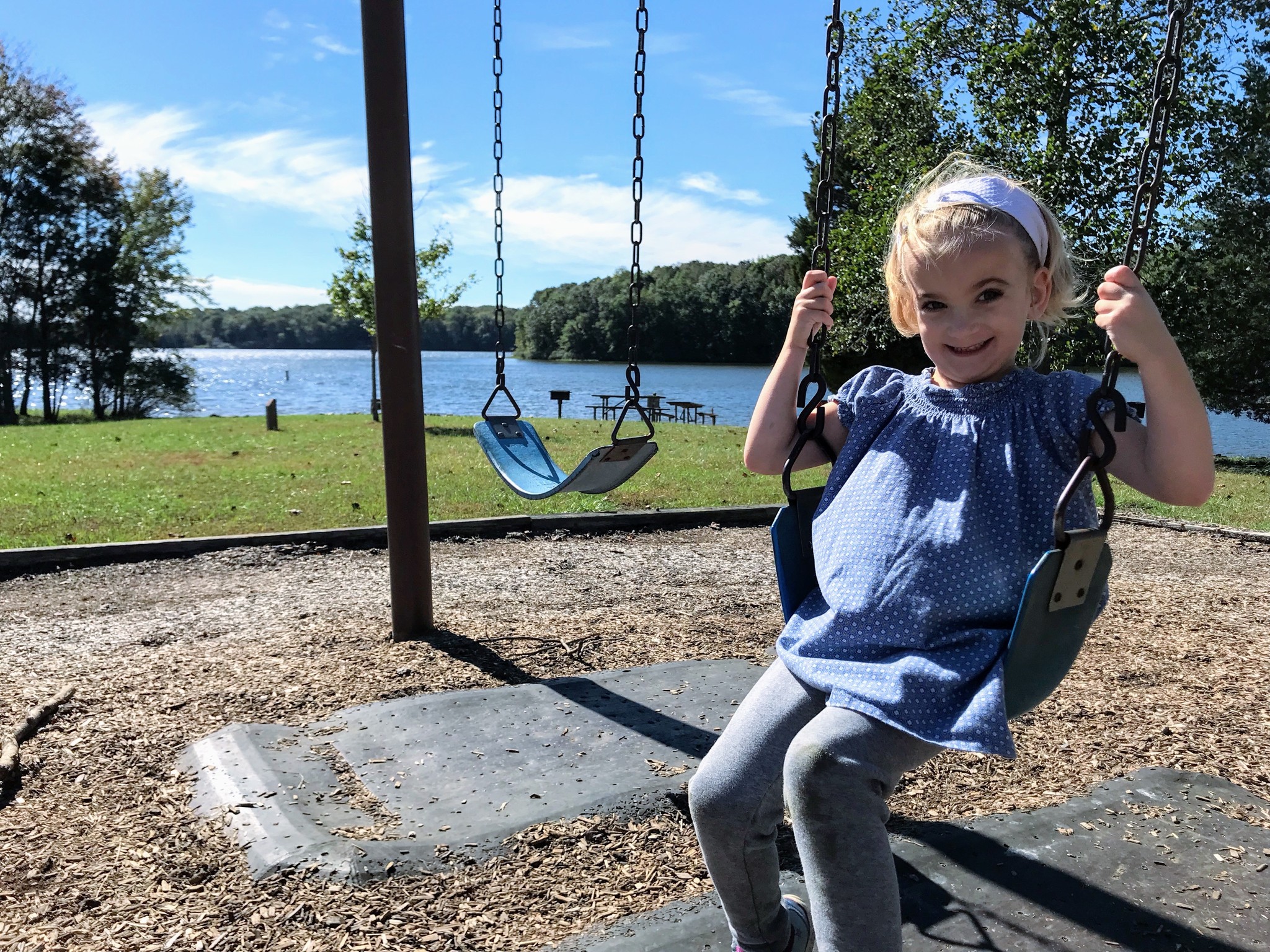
470, 2, 660, 499
767, 0, 1191, 728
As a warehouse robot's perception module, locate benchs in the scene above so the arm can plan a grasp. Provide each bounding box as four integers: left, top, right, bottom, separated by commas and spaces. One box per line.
654, 411, 675, 422
584, 405, 670, 422
697, 412, 719, 426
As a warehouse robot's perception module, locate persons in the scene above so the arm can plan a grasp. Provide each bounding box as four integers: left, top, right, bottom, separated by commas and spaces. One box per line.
685, 157, 1217, 951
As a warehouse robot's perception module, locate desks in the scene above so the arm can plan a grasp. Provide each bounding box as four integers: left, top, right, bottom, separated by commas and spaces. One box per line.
593, 393, 666, 420
665, 399, 705, 425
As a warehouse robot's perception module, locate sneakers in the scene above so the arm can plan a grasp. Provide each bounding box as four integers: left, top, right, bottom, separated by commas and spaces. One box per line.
731, 893, 815, 952
